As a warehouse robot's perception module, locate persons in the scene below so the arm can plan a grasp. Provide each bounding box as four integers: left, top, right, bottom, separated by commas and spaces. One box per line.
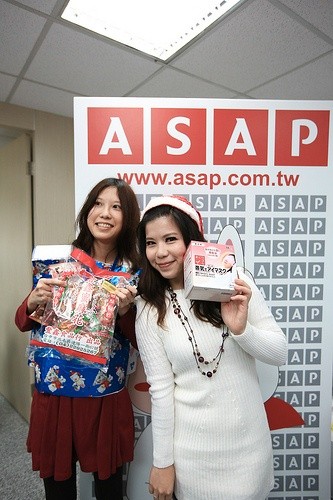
132, 195, 287, 500
14, 178, 141, 500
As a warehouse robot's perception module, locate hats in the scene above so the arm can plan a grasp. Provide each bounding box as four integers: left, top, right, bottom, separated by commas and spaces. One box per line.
139, 195, 203, 233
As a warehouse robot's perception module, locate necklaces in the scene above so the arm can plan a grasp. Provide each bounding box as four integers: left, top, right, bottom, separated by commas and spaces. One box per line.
165, 284, 229, 377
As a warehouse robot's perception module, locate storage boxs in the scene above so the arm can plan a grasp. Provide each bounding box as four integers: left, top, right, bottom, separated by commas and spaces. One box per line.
183, 240, 238, 303
